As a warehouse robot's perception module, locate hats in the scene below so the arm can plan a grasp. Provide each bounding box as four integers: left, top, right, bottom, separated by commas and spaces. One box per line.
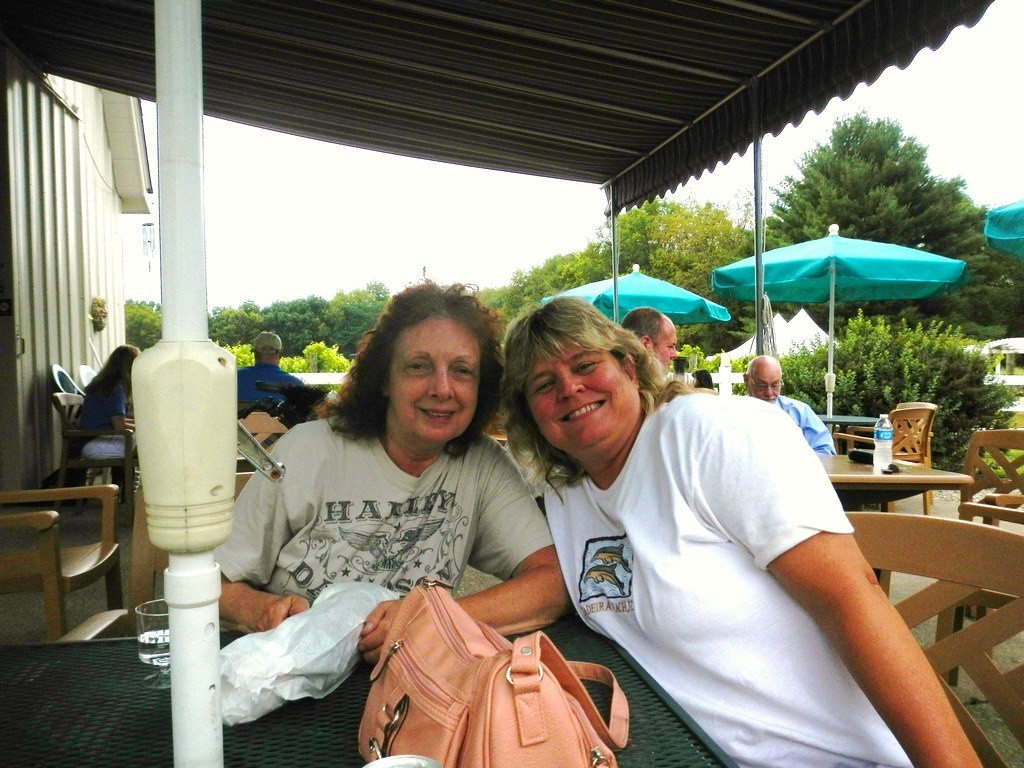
250, 332, 282, 353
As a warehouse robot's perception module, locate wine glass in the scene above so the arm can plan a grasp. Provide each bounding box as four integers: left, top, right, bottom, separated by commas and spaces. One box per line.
134, 598, 171, 689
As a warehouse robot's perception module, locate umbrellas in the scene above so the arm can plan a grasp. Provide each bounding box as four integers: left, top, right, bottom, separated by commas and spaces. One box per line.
711, 223, 967, 434
983, 199, 1024, 258
539, 265, 731, 326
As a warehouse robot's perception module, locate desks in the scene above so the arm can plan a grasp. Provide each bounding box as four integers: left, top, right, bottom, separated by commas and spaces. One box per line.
818, 412, 880, 426
0, 611, 739, 768
818, 452, 974, 505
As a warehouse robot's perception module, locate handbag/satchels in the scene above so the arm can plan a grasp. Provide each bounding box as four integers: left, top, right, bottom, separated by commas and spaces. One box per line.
357, 577, 630, 768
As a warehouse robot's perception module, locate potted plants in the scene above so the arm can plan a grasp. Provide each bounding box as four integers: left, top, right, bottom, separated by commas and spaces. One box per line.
92, 297, 109, 331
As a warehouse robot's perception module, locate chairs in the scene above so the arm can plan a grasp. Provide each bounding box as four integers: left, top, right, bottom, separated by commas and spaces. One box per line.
49, 364, 136, 516
834, 402, 938, 514
237, 411, 288, 451
0, 468, 131, 644
843, 511, 1024, 768
935, 429, 1024, 684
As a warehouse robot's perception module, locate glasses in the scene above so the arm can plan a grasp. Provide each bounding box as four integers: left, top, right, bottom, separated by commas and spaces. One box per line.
750, 377, 782, 390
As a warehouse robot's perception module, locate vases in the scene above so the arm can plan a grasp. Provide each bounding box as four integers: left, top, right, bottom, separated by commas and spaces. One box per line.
673, 356, 689, 375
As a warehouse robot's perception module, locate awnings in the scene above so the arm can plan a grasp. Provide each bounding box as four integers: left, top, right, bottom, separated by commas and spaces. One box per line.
0, 0, 993, 362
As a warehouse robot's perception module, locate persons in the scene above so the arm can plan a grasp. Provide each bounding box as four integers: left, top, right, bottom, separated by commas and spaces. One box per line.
498, 295, 984, 767
210, 279, 568, 662
620, 307, 678, 377
237, 330, 306, 403
691, 370, 714, 390
78, 344, 140, 458
743, 355, 838, 456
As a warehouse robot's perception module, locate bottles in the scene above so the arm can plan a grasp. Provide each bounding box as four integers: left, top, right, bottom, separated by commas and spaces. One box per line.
873, 414, 893, 471
